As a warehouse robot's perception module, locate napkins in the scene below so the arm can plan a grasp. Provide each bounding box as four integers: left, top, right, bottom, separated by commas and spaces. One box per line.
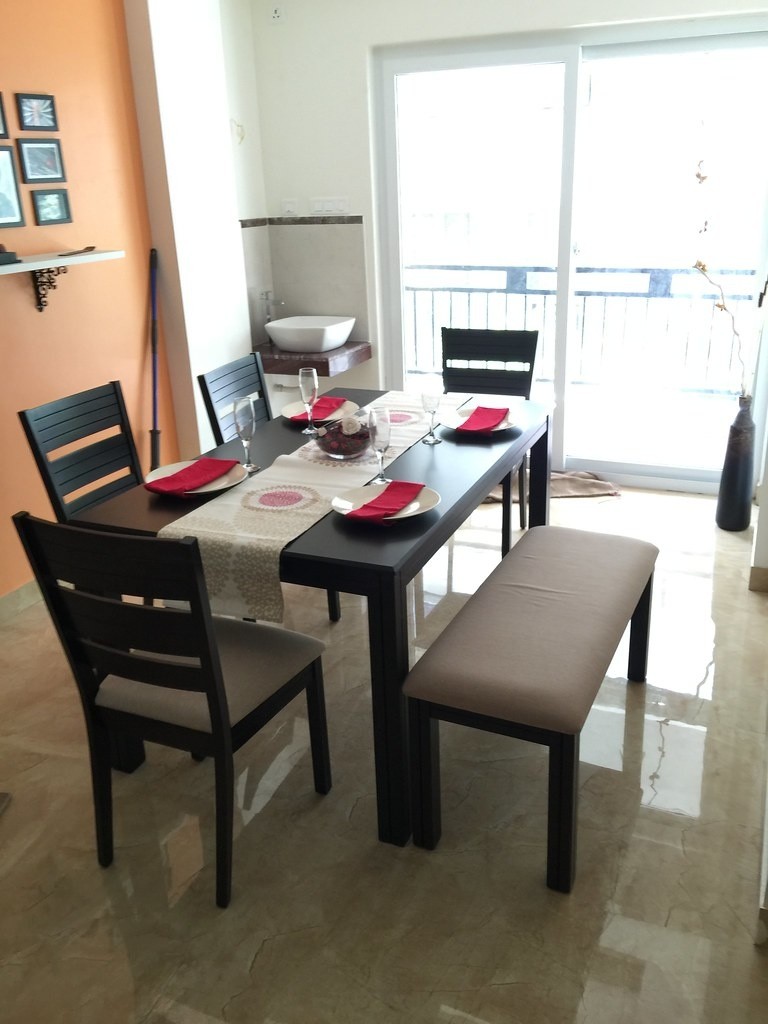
346, 480, 426, 522
143, 456, 240, 498
290, 396, 346, 424
456, 406, 510, 436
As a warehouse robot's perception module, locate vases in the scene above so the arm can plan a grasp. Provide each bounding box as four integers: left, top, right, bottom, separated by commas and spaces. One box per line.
715, 395, 755, 531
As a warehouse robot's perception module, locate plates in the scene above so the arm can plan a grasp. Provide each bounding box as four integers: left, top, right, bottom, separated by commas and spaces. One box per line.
281, 399, 360, 421
440, 409, 516, 431
144, 460, 248, 493
331, 483, 441, 519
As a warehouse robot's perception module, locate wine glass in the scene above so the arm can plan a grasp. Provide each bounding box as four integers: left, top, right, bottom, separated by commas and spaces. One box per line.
299, 368, 320, 434
421, 388, 442, 445
368, 407, 393, 485
234, 397, 261, 473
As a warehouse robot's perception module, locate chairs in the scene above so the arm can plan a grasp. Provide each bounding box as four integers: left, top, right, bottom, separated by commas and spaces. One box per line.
196, 352, 342, 623
441, 327, 538, 560
15, 380, 256, 623
10, 510, 332, 910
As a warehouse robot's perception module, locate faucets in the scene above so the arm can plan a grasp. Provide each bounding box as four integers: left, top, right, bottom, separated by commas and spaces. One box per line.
260, 290, 286, 323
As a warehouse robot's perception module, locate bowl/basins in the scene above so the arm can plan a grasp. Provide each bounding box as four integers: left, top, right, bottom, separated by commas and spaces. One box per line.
312, 417, 372, 460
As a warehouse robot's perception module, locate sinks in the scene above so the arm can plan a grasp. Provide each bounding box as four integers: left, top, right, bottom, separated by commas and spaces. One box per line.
264, 315, 356, 353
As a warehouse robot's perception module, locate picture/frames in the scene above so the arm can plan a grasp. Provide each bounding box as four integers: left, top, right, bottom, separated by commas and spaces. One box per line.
16, 92, 59, 131
0, 91, 10, 139
0, 145, 26, 228
31, 189, 73, 226
17, 138, 68, 184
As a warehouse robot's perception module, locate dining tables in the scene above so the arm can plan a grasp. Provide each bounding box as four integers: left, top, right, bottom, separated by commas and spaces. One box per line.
66, 386, 554, 847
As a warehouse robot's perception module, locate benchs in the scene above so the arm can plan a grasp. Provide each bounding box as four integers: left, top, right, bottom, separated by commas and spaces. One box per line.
401, 526, 660, 895
